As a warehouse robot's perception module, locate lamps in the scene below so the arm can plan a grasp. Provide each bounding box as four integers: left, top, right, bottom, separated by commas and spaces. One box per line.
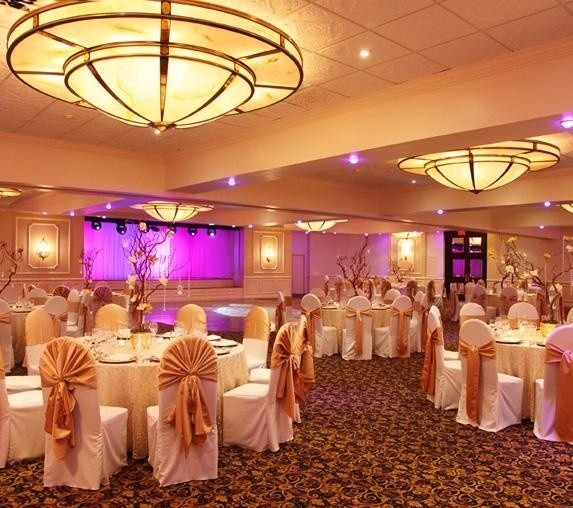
294, 219, 349, 232
91, 221, 101, 231
116, 223, 127, 234
5, 1, 304, 130
188, 224, 198, 237
207, 225, 216, 237
129, 200, 214, 223
397, 138, 561, 193
0, 186, 22, 199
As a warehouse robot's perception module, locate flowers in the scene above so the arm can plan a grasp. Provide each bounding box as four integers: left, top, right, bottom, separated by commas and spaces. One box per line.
335, 236, 372, 291
0, 241, 24, 297
117, 225, 193, 327
78, 248, 102, 289
499, 236, 573, 314
389, 257, 416, 280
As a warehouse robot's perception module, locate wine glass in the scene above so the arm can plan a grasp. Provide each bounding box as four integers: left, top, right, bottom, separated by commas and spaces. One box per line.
150, 322, 158, 337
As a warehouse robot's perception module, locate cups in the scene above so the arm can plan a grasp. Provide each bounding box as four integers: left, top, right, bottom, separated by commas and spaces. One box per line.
491, 317, 502, 335
85, 328, 116, 355
520, 321, 537, 347
175, 322, 203, 339
117, 328, 131, 341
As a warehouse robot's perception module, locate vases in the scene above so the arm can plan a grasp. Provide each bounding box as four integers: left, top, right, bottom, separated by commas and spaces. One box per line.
131, 331, 154, 351
541, 323, 558, 334
64, 289, 92, 337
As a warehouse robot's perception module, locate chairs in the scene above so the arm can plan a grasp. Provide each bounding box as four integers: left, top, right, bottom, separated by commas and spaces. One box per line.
478, 279, 484, 285
458, 319, 524, 430
0, 344, 44, 470
414, 287, 425, 300
23, 288, 50, 306
450, 286, 468, 322
0, 296, 15, 372
466, 283, 477, 303
43, 296, 68, 323
460, 302, 490, 329
309, 286, 326, 304
25, 309, 62, 375
429, 304, 459, 360
375, 296, 413, 359
421, 292, 431, 309
173, 303, 207, 336
426, 280, 443, 305
407, 281, 417, 303
241, 308, 270, 372
147, 336, 218, 488
534, 326, 573, 444
500, 289, 517, 318
52, 285, 70, 292
223, 321, 305, 453
471, 285, 496, 322
381, 280, 391, 296
249, 317, 306, 424
95, 303, 130, 330
323, 275, 329, 295
301, 293, 338, 355
507, 303, 540, 331
39, 337, 128, 491
95, 286, 112, 313
0, 346, 42, 392
385, 290, 400, 300
67, 290, 81, 326
274, 290, 306, 333
567, 308, 571, 322
334, 275, 343, 302
428, 309, 462, 408
342, 296, 373, 361
